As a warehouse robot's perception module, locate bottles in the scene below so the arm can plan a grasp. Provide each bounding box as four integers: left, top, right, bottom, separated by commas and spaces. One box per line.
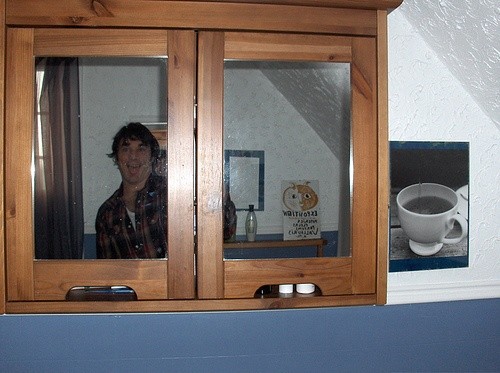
245, 204, 257, 242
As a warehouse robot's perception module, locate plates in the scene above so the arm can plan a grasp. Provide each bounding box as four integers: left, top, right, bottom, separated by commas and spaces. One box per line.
455, 183, 469, 221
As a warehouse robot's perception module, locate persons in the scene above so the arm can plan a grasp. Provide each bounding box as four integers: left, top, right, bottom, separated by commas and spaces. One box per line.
94, 120, 238, 259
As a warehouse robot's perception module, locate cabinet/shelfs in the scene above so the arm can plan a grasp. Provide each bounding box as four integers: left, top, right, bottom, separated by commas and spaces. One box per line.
0, 0, 404, 314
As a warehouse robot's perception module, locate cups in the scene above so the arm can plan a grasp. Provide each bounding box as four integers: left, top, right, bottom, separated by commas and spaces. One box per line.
296, 284, 315, 294
279, 284, 293, 293
395, 182, 468, 257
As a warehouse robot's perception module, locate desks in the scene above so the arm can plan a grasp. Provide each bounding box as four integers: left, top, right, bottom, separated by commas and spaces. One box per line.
223, 238, 328, 257
